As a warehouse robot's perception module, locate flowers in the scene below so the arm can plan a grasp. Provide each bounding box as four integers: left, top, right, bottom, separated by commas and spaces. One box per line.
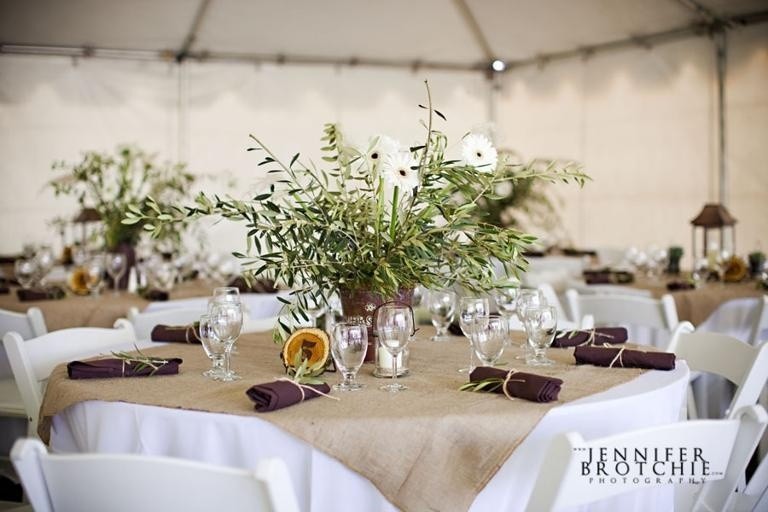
120, 80, 594, 349
42, 139, 192, 249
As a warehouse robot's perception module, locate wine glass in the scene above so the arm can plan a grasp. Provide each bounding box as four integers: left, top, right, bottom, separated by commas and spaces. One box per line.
375, 304, 414, 396
328, 320, 370, 393
427, 286, 558, 380
199, 283, 249, 385
622, 245, 768, 292
11, 242, 129, 300
